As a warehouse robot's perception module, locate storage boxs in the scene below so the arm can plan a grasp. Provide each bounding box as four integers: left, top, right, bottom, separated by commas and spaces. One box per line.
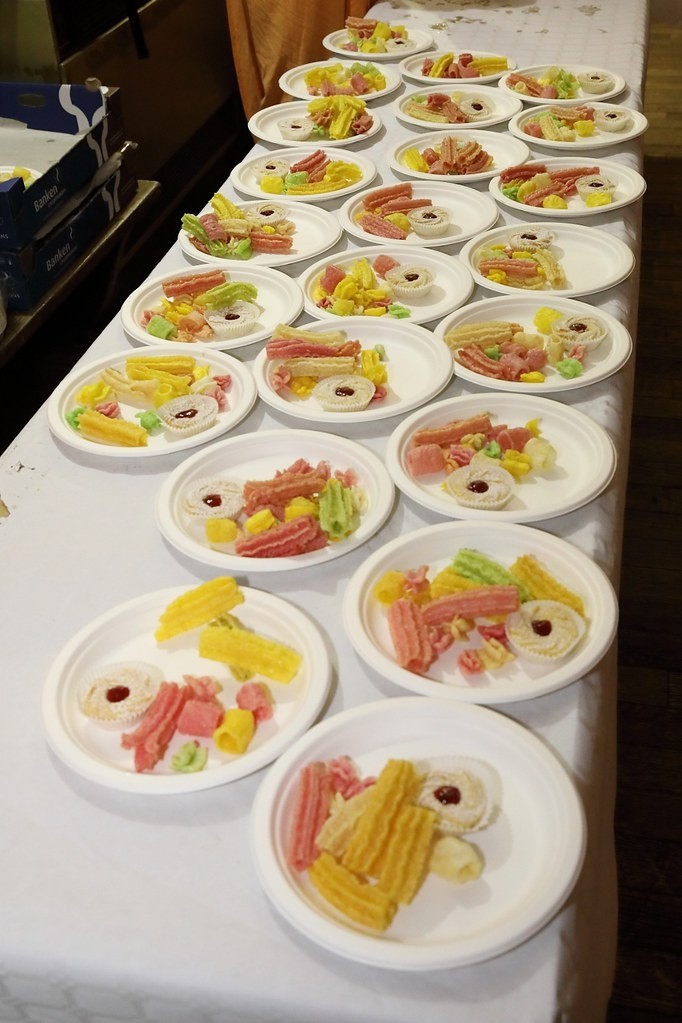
0, 141, 139, 314
0, 79, 125, 254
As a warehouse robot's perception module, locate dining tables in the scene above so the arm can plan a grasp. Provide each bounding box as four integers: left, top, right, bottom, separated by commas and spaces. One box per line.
0, 0, 652, 1023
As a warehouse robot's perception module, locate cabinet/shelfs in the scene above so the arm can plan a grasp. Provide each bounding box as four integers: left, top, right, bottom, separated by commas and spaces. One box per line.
0, 179, 163, 458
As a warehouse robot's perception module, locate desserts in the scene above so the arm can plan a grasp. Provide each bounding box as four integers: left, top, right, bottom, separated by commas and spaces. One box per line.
0, 166, 36, 190
70, 18, 627, 932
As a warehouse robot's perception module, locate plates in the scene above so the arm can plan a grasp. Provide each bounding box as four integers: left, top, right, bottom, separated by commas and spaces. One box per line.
48, 346, 258, 458
433, 295, 633, 393
154, 429, 396, 573
386, 392, 618, 523
459, 222, 636, 298
44, 585, 331, 795
253, 316, 454, 423
122, 263, 305, 350
178, 23, 648, 266
342, 520, 620, 704
297, 245, 475, 325
247, 698, 588, 975
